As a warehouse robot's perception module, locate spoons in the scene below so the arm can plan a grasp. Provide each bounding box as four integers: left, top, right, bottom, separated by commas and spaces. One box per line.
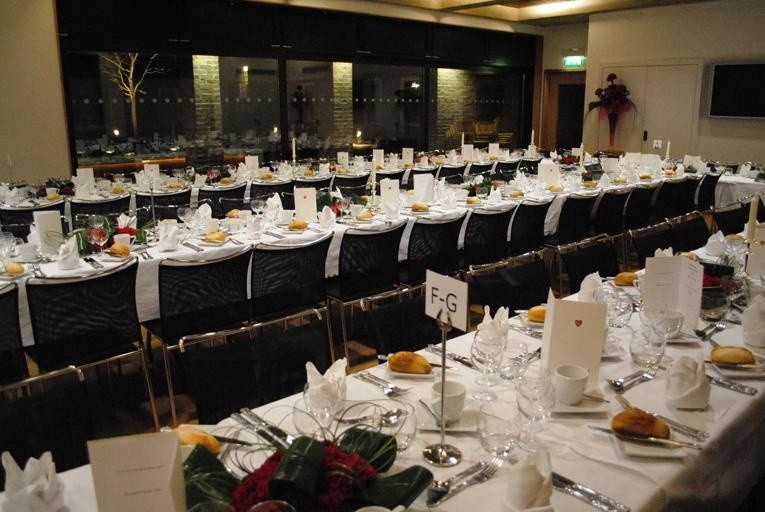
425, 461, 488, 501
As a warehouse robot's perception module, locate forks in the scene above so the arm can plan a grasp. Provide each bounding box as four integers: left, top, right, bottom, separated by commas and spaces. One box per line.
426, 453, 504, 507
616, 395, 711, 441
615, 361, 657, 392
354, 367, 411, 396
693, 319, 728, 342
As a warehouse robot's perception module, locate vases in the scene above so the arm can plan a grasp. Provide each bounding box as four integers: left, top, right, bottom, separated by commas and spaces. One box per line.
604, 111, 620, 153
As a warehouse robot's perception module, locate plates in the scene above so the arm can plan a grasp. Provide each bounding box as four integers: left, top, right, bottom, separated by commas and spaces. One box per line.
551, 377, 612, 415
520, 309, 548, 327
709, 349, 765, 381
415, 398, 482, 434
386, 353, 436, 380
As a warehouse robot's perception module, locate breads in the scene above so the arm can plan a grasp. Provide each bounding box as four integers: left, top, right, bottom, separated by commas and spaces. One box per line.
167, 183, 181, 188
528, 306, 546, 323
613, 409, 669, 438
356, 213, 371, 220
639, 175, 652, 180
47, 194, 60, 200
725, 235, 743, 240
581, 181, 597, 187
614, 178, 627, 183
550, 184, 563, 191
387, 351, 431, 375
109, 243, 128, 256
205, 231, 224, 240
175, 427, 218, 454
465, 197, 480, 203
510, 191, 523, 196
113, 187, 125, 194
411, 203, 428, 212
225, 210, 240, 217
288, 220, 308, 229
681, 253, 699, 260
221, 178, 230, 184
712, 347, 754, 363
664, 171, 675, 176
4, 263, 24, 274
614, 271, 638, 287
305, 171, 314, 176
489, 155, 499, 161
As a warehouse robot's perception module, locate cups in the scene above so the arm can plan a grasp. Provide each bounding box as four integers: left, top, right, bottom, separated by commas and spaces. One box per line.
292, 379, 341, 439
554, 362, 589, 406
428, 381, 467, 421
478, 400, 523, 457
594, 289, 685, 368
113, 234, 137, 255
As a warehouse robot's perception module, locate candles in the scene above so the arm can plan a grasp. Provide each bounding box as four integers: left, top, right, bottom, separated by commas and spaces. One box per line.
291, 138, 295, 160
462, 132, 464, 146
531, 129, 534, 144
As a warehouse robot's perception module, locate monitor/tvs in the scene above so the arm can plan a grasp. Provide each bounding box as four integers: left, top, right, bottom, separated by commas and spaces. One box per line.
707, 62, 765, 121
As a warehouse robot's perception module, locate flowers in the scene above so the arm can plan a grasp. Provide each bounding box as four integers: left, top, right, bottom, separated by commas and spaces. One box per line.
588, 73, 633, 113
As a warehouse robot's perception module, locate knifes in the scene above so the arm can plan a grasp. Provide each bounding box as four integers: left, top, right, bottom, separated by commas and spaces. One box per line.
552, 473, 630, 512
588, 423, 705, 453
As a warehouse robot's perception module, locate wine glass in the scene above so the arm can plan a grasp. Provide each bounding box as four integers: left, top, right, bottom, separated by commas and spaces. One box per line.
0, 231, 26, 277
469, 330, 525, 386
13, 152, 332, 200
712, 161, 763, 180
174, 200, 285, 238
337, 154, 675, 223
517, 364, 555, 466
72, 214, 109, 263
